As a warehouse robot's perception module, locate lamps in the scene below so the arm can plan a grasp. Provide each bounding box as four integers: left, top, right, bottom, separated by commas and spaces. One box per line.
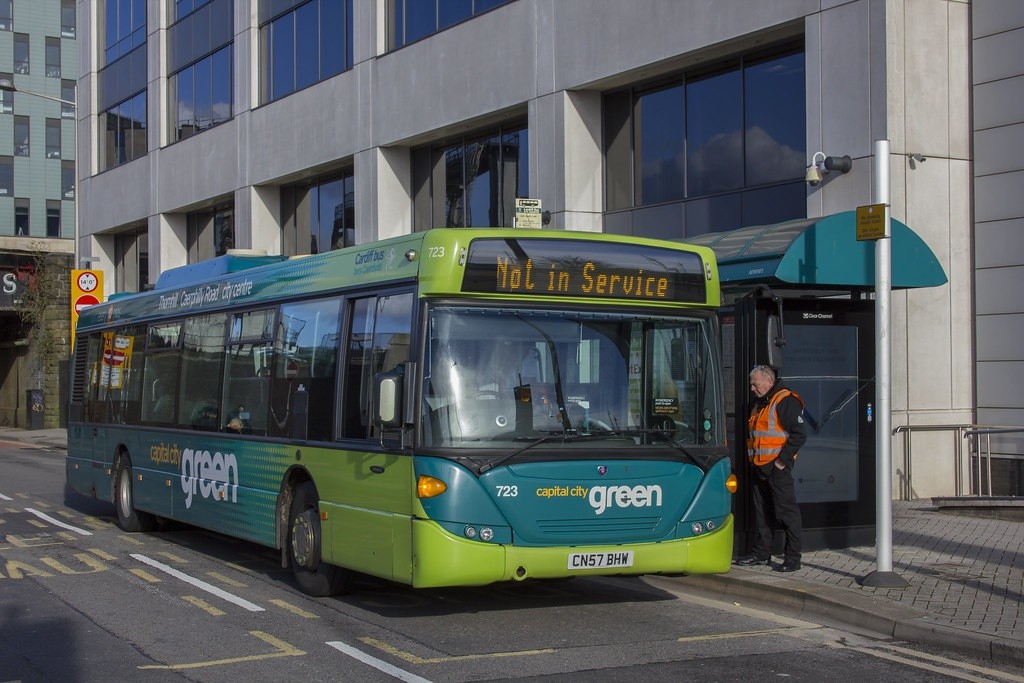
804, 151, 830, 186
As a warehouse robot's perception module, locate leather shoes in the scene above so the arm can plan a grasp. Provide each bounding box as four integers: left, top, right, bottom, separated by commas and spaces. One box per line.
779, 561, 801, 572
736, 553, 771, 566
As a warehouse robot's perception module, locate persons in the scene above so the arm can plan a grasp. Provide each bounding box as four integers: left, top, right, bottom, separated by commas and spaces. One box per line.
226, 404, 252, 433
192, 406, 217, 430
153, 377, 175, 421
736, 365, 808, 572
227, 417, 243, 431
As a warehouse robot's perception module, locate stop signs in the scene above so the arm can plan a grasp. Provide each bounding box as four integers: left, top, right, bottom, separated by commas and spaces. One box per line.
74, 295, 100, 318
103, 349, 125, 366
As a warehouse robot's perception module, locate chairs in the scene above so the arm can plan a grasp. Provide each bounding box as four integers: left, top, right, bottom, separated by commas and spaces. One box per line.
146, 366, 292, 438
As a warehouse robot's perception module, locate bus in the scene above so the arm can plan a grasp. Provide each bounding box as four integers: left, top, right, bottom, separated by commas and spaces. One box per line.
66, 227, 789, 598
